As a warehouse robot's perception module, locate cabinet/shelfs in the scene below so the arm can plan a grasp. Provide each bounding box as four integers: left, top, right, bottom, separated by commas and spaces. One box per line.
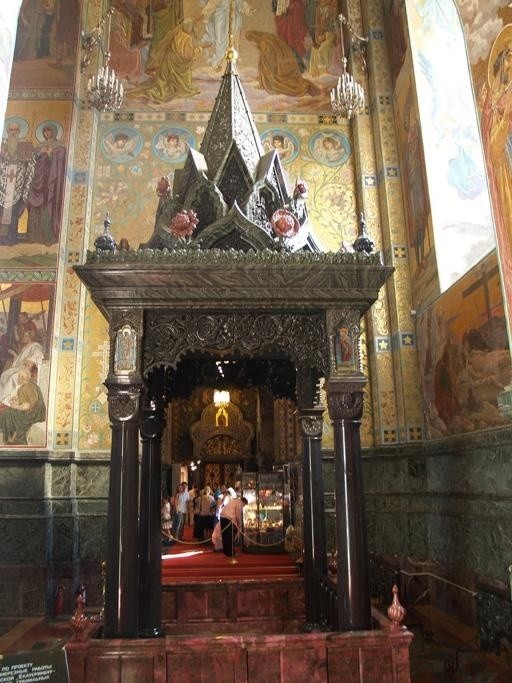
241, 471, 285, 553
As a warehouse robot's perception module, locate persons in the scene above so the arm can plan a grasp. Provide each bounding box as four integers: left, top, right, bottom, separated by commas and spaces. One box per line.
154, 129, 190, 161
103, 131, 140, 157
402, 102, 428, 280
263, 131, 295, 162
1, 311, 48, 446
22, 122, 66, 247
319, 135, 343, 164
0, 121, 37, 246
158, 478, 250, 558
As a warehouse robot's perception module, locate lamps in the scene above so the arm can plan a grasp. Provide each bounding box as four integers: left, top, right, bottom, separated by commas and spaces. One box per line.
80, 6, 124, 115
330, 13, 369, 121
